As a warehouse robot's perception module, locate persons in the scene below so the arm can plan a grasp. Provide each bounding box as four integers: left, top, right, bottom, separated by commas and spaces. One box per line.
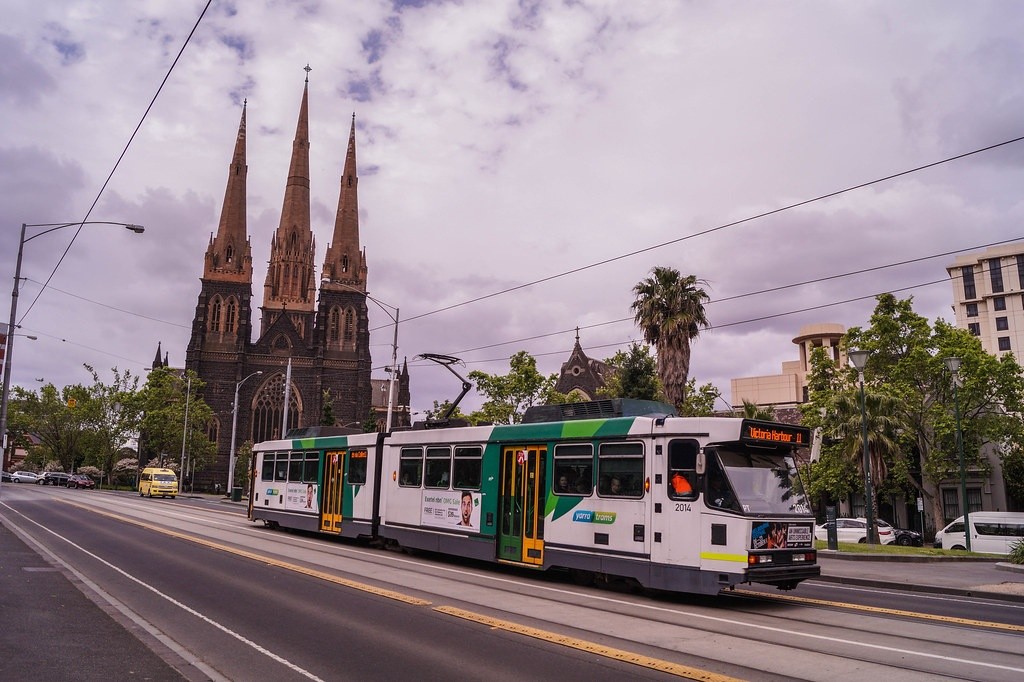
556, 476, 569, 493
304, 484, 313, 509
767, 523, 787, 549
604, 475, 625, 495
456, 491, 473, 526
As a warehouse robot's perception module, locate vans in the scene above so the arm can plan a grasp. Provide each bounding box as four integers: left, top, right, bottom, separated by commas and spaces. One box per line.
139, 468, 178, 499
933, 509, 1024, 554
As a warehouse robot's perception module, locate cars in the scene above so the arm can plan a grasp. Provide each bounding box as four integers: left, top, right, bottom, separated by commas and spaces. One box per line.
11, 471, 39, 484
815, 517, 897, 545
860, 517, 923, 548
45, 473, 71, 486
36, 472, 48, 484
2, 472, 12, 483
67, 475, 95, 491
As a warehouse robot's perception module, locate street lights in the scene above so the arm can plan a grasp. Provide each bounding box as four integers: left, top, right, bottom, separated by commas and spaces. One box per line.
848, 350, 874, 545
144, 368, 191, 494
321, 278, 399, 433
0, 222, 145, 484
160, 454, 168, 468
343, 422, 361, 427
227, 371, 263, 497
944, 355, 972, 553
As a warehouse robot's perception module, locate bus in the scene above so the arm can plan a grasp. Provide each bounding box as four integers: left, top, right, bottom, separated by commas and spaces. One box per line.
250, 352, 823, 599
